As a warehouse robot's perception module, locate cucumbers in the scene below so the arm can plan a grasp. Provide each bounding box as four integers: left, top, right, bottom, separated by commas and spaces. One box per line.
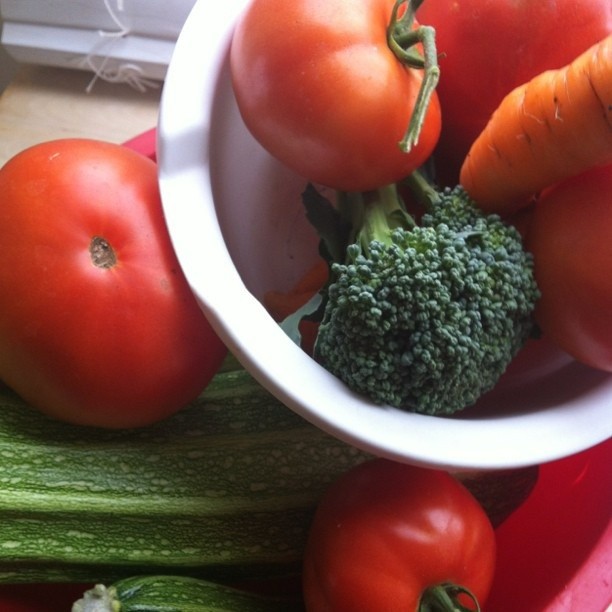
71, 570, 291, 609
0, 373, 359, 582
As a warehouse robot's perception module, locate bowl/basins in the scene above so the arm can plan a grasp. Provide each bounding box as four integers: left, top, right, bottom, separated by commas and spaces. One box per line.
157, 0, 612, 480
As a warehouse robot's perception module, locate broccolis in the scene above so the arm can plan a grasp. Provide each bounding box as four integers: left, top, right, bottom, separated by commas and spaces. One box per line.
304, 171, 545, 417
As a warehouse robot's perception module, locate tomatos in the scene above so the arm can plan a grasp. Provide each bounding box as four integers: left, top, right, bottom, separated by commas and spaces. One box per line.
227, 1, 443, 194
513, 175, 612, 381
0, 139, 229, 432
304, 458, 497, 611
414, 3, 612, 177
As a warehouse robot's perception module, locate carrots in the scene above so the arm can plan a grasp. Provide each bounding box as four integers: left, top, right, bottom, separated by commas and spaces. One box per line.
459, 30, 611, 211
